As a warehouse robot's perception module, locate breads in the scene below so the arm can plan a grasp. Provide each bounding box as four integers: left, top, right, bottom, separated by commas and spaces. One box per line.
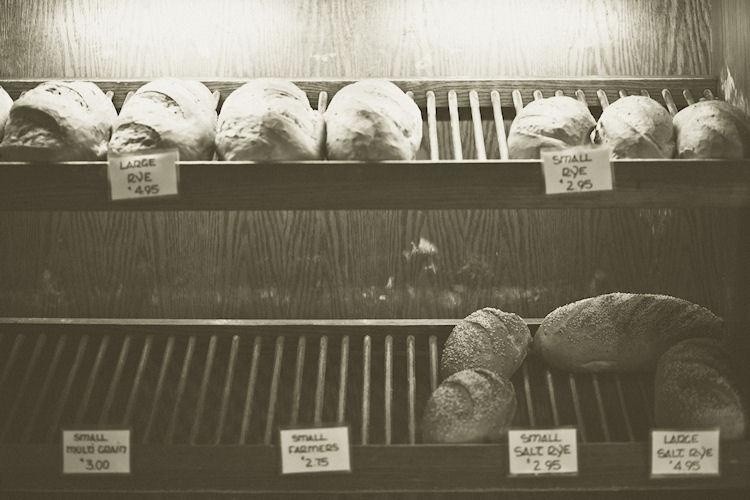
0, 78, 423, 162
421, 366, 518, 445
596, 95, 675, 159
673, 99, 750, 158
531, 293, 728, 376
506, 97, 596, 160
440, 305, 533, 383
654, 336, 744, 443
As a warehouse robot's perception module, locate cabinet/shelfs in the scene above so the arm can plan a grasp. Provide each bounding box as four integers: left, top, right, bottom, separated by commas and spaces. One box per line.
0, 77, 750, 500
